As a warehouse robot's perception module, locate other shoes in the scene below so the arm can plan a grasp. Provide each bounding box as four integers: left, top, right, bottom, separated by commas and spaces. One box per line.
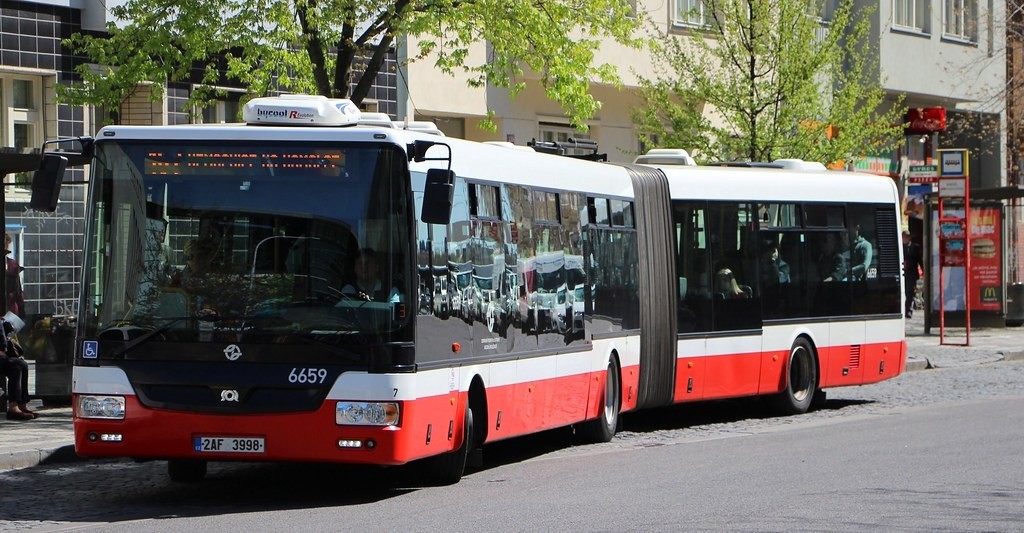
26, 411, 40, 418
6, 410, 33, 419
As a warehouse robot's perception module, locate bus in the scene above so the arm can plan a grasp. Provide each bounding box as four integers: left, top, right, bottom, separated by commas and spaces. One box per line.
29, 94, 906, 484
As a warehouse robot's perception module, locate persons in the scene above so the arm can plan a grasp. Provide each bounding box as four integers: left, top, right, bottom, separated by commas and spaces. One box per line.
833, 219, 873, 282
711, 245, 790, 301
902, 231, 924, 318
341, 248, 404, 310
171, 239, 230, 316
0, 233, 40, 421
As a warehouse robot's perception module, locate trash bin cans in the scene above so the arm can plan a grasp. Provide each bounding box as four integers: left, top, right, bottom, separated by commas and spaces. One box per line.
33, 314, 78, 406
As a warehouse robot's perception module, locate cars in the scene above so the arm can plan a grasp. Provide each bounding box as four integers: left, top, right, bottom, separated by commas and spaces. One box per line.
418, 251, 596, 345
